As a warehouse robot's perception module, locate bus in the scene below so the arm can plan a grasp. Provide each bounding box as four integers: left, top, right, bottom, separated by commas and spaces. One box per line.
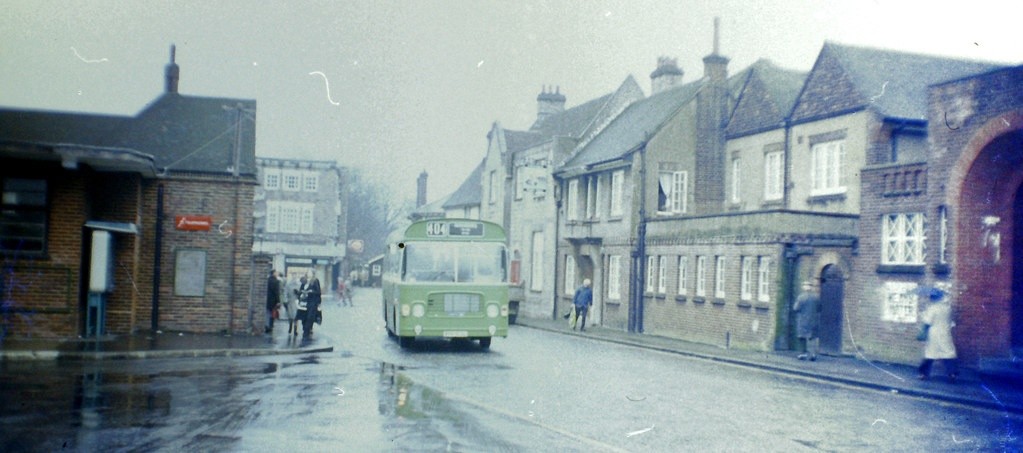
381, 218, 508, 350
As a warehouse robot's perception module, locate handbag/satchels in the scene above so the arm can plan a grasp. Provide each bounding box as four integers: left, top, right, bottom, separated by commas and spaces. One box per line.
316, 308, 323, 324
567, 305, 576, 330
563, 307, 574, 319
917, 323, 930, 341
271, 309, 279, 318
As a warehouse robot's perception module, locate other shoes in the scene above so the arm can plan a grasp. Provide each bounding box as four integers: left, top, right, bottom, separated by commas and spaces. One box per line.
809, 355, 816, 361
797, 353, 806, 359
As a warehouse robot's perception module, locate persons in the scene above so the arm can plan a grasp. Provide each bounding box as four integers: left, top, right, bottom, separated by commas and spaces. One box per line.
794, 282, 822, 360
337, 276, 353, 306
266, 267, 322, 337
917, 289, 957, 383
573, 278, 592, 330
